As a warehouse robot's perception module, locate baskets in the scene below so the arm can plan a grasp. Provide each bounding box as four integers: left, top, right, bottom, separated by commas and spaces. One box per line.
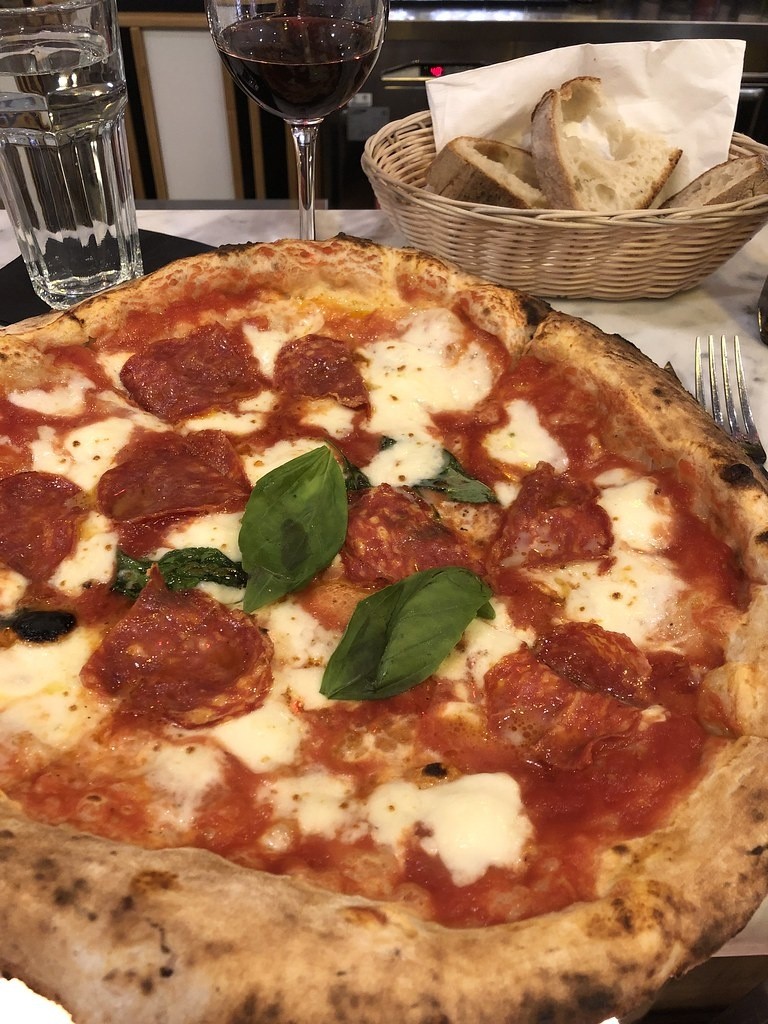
360, 109, 768, 298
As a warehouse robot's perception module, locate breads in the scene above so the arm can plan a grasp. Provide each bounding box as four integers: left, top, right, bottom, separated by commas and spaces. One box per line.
424, 76, 768, 212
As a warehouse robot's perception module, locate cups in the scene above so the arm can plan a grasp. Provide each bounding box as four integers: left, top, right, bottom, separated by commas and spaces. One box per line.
0, 1, 143, 310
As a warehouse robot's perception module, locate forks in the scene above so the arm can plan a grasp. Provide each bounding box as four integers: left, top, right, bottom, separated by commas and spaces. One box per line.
692, 334, 768, 480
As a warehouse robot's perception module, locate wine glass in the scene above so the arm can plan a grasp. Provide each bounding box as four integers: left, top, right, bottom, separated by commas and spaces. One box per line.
207, 0, 390, 241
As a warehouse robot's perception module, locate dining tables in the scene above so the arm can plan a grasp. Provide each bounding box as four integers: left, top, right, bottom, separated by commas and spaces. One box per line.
0, 210, 768, 1024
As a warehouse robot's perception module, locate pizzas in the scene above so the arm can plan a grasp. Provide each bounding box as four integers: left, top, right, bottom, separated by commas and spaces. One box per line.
0, 233, 768, 1023
